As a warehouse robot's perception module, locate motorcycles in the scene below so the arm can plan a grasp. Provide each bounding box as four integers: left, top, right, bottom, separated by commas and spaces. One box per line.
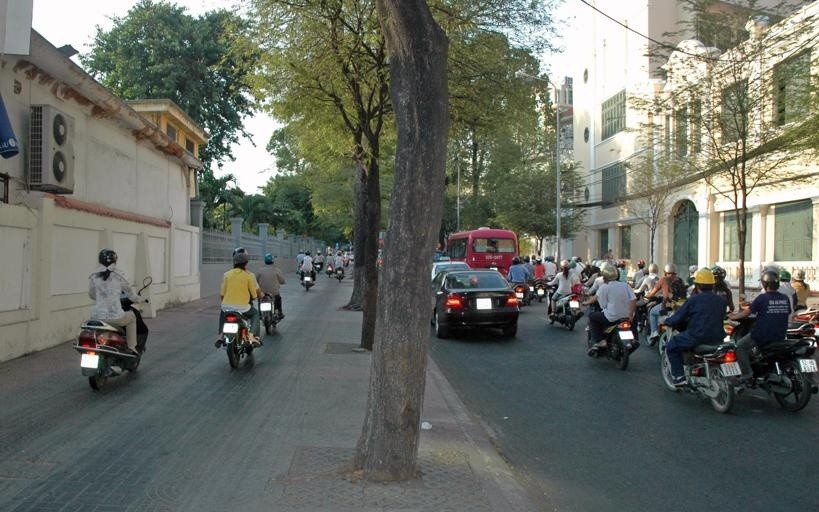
584, 303, 640, 371
72, 275, 152, 392
635, 292, 688, 356
295, 247, 350, 291
541, 280, 583, 332
512, 271, 557, 307
660, 324, 742, 414
216, 308, 261, 368
259, 290, 284, 334
725, 303, 818, 413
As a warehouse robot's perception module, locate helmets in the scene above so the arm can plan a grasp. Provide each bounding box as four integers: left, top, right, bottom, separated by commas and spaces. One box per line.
511, 254, 807, 284
299, 249, 343, 254
100, 249, 118, 272
233, 246, 249, 266
265, 252, 275, 265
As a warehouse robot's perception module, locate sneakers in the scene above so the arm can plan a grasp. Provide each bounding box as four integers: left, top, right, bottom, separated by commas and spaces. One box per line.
673, 375, 689, 387
127, 348, 142, 356
593, 340, 608, 347
250, 334, 262, 346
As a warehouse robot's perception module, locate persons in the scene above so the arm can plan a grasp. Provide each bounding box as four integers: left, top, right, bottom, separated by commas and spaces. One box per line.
507, 254, 809, 348
87, 247, 146, 356
661, 266, 726, 387
300, 250, 315, 274
314, 250, 325, 266
296, 250, 306, 271
728, 272, 790, 380
213, 253, 262, 348
327, 252, 335, 273
254, 253, 286, 321
221, 248, 265, 345
334, 251, 345, 275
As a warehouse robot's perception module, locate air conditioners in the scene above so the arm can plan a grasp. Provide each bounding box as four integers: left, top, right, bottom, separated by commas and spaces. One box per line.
25, 99, 77, 196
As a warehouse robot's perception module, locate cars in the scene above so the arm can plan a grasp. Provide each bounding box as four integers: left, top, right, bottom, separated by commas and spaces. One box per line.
432, 262, 471, 279
430, 272, 520, 339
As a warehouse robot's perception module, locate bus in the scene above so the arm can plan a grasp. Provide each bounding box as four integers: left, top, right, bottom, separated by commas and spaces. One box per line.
447, 227, 518, 277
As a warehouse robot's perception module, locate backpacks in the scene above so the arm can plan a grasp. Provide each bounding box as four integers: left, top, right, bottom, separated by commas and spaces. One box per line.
667, 278, 688, 302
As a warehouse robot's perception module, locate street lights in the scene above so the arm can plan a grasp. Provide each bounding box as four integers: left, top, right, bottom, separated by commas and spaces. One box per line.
514, 69, 562, 272
